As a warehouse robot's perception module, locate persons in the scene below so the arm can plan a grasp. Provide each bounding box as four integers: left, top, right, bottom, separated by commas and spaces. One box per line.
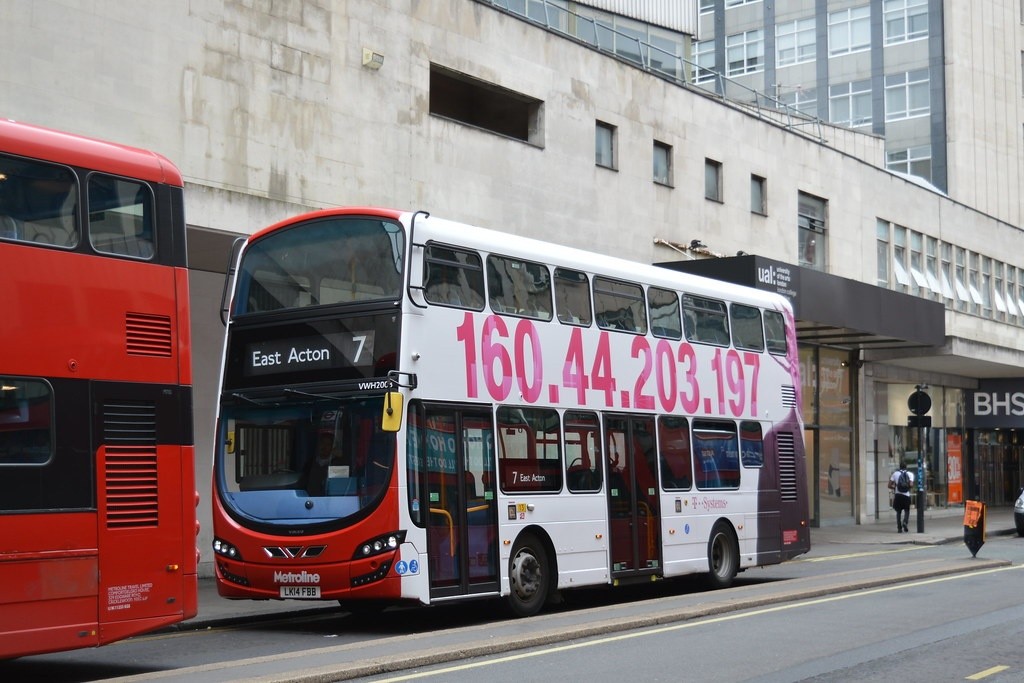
888, 462, 914, 532
296, 432, 334, 496
605, 308, 637, 332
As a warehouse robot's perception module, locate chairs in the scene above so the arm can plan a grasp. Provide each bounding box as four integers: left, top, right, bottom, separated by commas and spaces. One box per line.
539, 458, 685, 508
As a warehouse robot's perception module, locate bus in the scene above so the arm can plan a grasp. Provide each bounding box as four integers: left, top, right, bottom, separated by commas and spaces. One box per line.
210, 206, 812, 618
0, 119, 200, 660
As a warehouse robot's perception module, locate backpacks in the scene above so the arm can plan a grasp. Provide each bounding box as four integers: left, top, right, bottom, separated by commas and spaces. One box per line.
895, 470, 910, 492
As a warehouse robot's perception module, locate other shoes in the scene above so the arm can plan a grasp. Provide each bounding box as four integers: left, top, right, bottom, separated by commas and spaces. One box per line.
898, 526, 902, 533
902, 522, 908, 532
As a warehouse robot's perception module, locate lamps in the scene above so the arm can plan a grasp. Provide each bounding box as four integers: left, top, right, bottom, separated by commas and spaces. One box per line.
363, 47, 384, 70
690, 239, 707, 252
737, 249, 749, 256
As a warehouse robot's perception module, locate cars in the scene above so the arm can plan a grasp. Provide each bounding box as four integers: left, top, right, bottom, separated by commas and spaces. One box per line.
1014, 488, 1024, 536
